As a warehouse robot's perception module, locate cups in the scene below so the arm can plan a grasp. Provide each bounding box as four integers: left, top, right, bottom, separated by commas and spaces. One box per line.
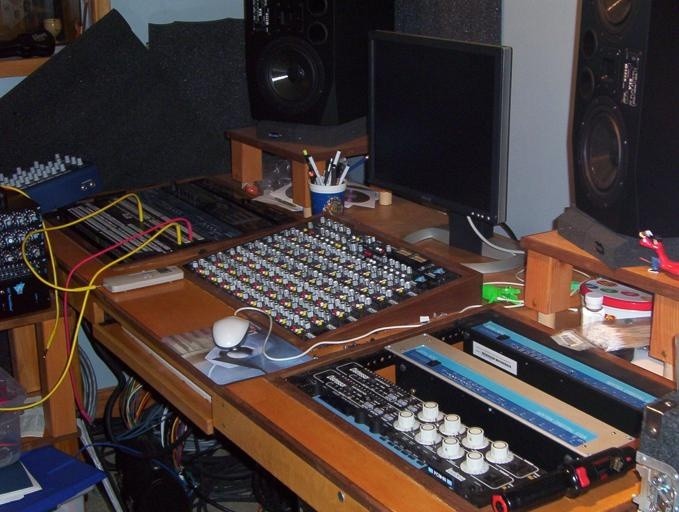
308, 176, 346, 219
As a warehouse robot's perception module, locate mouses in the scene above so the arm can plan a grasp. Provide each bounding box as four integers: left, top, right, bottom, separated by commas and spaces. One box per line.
211, 315, 252, 350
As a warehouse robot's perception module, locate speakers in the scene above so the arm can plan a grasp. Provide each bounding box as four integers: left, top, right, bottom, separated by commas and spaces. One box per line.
556, 0, 679, 268
242, 0, 396, 148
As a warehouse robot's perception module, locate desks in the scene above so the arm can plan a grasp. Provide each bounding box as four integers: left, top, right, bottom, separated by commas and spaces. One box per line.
1, 292, 81, 458
40, 127, 679, 512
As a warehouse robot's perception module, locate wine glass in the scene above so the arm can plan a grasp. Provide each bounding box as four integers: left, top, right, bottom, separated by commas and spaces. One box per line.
42, 19, 61, 44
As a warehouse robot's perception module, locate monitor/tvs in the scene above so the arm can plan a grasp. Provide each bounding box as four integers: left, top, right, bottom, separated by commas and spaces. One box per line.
362, 28, 525, 275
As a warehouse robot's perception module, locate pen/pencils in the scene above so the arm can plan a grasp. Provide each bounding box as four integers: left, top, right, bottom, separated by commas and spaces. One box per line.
304, 149, 369, 185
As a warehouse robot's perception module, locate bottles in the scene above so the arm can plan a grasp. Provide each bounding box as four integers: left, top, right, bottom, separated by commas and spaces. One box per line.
581, 292, 605, 333
